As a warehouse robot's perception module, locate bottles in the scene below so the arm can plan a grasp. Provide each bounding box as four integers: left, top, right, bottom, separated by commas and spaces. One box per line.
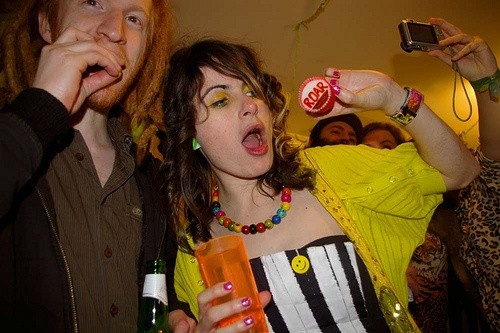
136, 259, 169, 333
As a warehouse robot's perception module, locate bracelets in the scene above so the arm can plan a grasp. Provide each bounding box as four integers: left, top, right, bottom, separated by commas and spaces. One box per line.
469, 68, 500, 94
384, 87, 424, 126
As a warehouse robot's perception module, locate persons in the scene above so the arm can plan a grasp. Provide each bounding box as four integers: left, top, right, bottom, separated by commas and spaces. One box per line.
310, 113, 363, 148
1, 0, 198, 333
158, 37, 481, 333
425, 17, 500, 333
358, 121, 450, 333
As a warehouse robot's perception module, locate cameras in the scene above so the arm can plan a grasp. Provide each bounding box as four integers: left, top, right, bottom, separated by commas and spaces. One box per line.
398, 20, 448, 52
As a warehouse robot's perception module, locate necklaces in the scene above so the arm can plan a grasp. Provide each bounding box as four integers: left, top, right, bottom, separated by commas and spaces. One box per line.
212, 183, 292, 235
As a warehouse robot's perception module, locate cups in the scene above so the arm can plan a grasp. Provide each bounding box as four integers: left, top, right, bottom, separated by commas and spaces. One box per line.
195, 235, 269, 333
